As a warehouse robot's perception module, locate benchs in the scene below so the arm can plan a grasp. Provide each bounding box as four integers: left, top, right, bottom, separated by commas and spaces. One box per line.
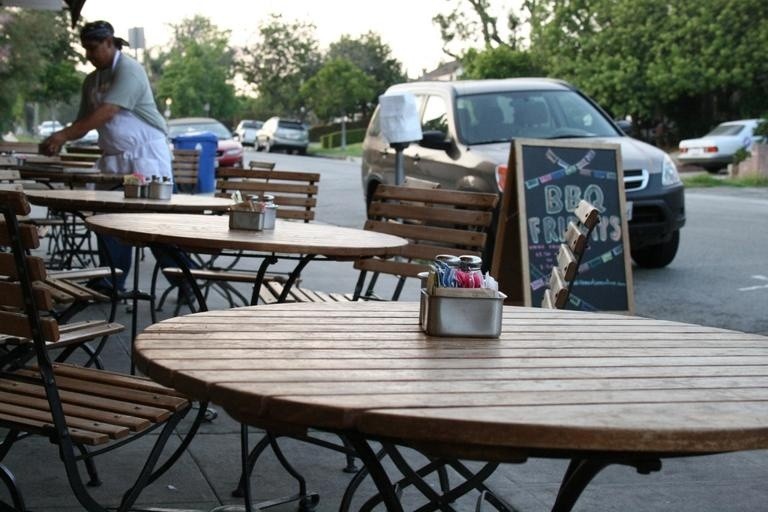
0, 136, 519, 361
0, 191, 194, 512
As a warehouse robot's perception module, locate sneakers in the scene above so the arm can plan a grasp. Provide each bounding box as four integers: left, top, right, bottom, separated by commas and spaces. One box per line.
177, 283, 196, 305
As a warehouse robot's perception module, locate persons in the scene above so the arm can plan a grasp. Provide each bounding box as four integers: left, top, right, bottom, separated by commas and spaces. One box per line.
42, 21, 195, 305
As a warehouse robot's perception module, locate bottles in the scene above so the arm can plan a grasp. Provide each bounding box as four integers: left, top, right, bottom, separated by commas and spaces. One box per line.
151, 174, 171, 183
437, 252, 484, 288
246, 193, 275, 206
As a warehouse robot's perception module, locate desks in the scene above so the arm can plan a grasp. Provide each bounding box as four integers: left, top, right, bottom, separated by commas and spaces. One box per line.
133, 296, 766, 512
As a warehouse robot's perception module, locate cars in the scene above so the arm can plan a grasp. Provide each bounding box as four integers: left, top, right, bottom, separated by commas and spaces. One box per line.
162, 110, 247, 168
231, 118, 261, 146
36, 119, 65, 138
676, 116, 766, 177
70, 127, 99, 146
609, 120, 633, 138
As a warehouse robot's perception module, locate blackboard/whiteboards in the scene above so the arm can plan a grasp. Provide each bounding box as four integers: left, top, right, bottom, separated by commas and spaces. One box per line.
514, 137, 635, 315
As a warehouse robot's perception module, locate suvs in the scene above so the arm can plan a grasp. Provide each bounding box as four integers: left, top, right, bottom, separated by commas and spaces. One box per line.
251, 114, 310, 156
355, 75, 696, 276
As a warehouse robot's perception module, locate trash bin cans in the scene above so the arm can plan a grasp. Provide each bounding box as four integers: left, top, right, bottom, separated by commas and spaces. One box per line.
172, 133, 218, 194
